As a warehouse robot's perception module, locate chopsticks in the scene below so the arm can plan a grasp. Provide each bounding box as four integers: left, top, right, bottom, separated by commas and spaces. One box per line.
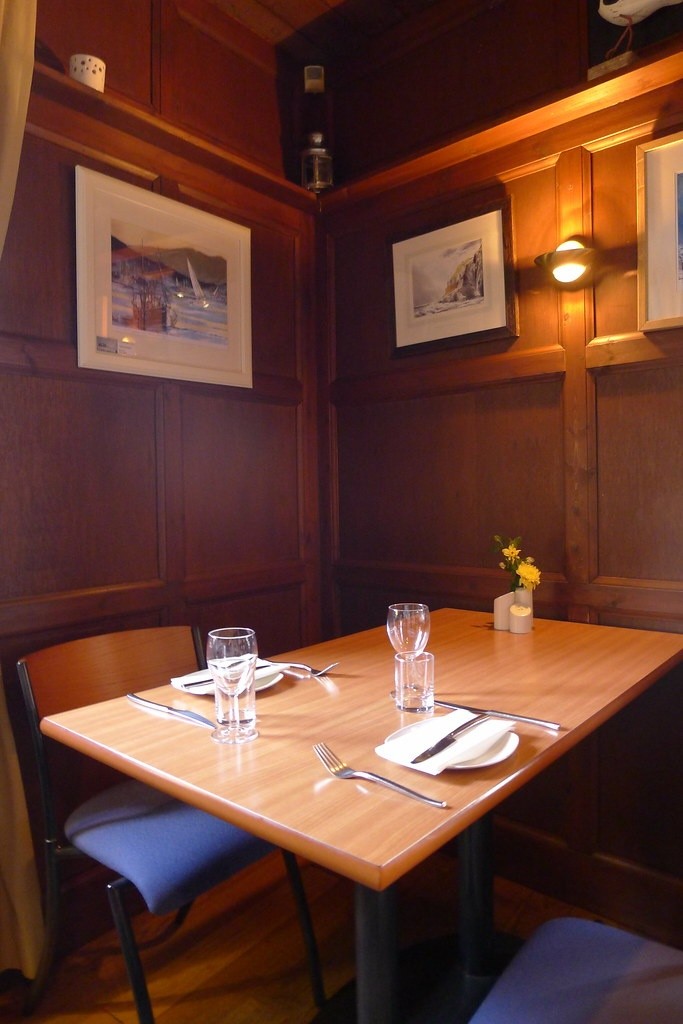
184, 665, 270, 687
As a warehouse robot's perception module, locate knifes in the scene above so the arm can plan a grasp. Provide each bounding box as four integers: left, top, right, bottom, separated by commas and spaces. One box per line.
410, 713, 491, 764
434, 696, 561, 731
126, 693, 216, 730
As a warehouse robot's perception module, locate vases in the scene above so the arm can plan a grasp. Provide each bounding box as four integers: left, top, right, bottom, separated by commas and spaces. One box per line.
512, 585, 533, 607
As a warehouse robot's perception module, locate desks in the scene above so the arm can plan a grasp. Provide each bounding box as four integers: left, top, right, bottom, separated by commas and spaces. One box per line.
41, 609, 683, 1024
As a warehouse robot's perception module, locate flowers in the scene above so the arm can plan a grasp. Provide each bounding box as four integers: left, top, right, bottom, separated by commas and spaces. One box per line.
493, 534, 541, 593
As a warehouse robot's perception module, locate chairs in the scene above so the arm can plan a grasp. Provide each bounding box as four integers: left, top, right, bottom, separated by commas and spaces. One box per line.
0, 625, 328, 1024
471, 916, 683, 1024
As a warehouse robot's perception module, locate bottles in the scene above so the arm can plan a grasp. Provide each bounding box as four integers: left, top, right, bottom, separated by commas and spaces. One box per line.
301, 132, 334, 190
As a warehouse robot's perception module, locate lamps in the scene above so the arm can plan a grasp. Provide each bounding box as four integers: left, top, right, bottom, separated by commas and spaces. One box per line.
533, 240, 599, 289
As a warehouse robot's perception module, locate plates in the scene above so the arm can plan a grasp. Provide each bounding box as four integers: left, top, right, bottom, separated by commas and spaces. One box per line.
170, 652, 284, 695
384, 716, 519, 769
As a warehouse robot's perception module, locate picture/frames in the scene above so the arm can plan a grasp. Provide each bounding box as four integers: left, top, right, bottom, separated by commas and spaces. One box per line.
384, 194, 519, 356
636, 131, 682, 333
75, 165, 252, 389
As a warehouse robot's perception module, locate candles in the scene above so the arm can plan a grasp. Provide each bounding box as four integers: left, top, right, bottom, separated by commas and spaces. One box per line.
493, 591, 533, 633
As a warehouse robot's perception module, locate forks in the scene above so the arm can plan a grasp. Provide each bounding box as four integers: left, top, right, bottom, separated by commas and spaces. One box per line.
312, 741, 447, 808
271, 661, 340, 677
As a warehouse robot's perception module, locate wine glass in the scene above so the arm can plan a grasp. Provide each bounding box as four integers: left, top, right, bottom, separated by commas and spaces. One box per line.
206, 627, 259, 743
386, 602, 431, 702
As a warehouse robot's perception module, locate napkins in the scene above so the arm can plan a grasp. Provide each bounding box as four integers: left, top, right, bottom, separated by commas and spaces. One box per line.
374, 708, 517, 776
169, 652, 291, 696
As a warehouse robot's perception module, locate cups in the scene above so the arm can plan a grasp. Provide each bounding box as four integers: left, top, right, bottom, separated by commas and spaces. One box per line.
69, 54, 106, 93
394, 651, 435, 713
215, 656, 257, 725
